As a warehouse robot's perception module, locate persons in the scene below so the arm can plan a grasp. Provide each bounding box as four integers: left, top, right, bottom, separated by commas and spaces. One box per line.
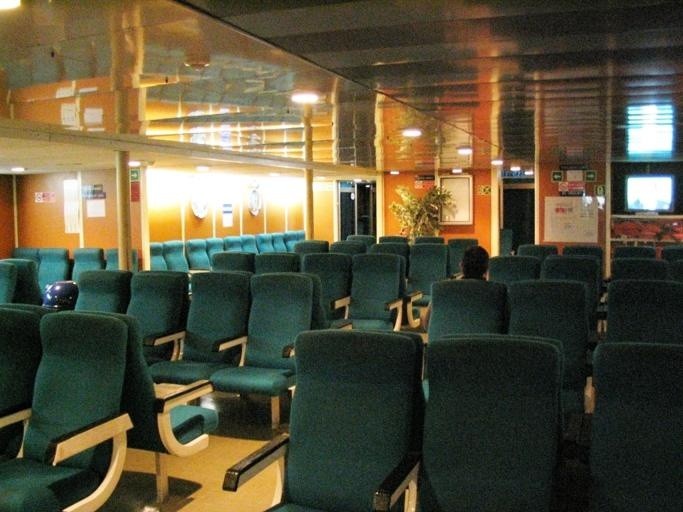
458, 246, 490, 280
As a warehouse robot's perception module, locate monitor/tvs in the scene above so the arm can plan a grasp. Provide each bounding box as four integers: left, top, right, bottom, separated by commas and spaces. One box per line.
624, 173, 676, 217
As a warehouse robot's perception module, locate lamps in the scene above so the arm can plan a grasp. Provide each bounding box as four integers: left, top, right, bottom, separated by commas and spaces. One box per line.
2, 0, 19, 11
11, 166, 26, 172
